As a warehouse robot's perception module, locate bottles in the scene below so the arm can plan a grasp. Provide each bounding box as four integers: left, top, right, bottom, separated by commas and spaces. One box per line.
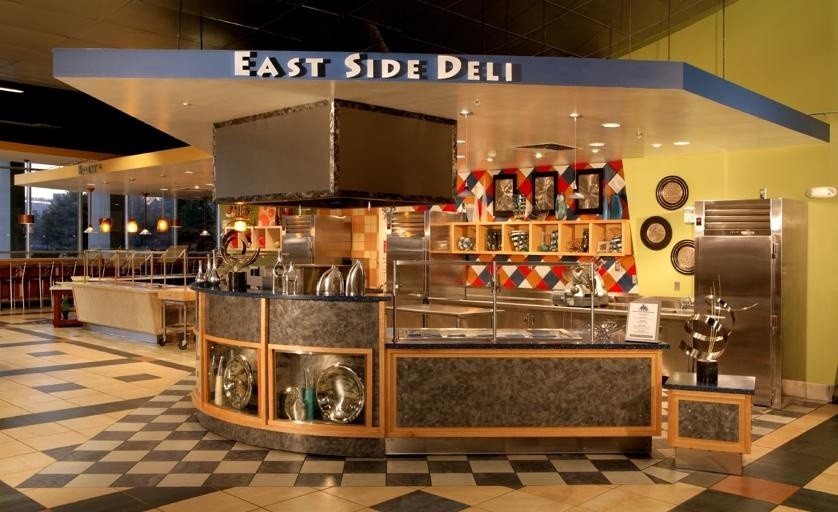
194, 247, 220, 287
303, 366, 314, 422
270, 246, 300, 296
208, 346, 223, 410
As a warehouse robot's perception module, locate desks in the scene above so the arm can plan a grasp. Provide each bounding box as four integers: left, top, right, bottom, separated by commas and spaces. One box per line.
389, 302, 505, 328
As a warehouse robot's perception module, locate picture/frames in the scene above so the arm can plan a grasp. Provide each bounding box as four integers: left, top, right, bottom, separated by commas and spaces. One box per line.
492, 174, 518, 217
532, 171, 557, 215
574, 170, 603, 214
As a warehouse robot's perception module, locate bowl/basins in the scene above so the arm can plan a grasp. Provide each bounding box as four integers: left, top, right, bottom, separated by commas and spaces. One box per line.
314, 363, 366, 424
223, 353, 254, 410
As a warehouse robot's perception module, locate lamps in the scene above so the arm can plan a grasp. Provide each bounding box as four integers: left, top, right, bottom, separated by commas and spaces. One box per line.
225, 203, 252, 231
19, 156, 35, 224
83, 184, 210, 239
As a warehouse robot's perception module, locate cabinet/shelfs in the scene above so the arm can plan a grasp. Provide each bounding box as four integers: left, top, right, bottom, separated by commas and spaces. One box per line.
449, 218, 632, 259
223, 224, 282, 254
